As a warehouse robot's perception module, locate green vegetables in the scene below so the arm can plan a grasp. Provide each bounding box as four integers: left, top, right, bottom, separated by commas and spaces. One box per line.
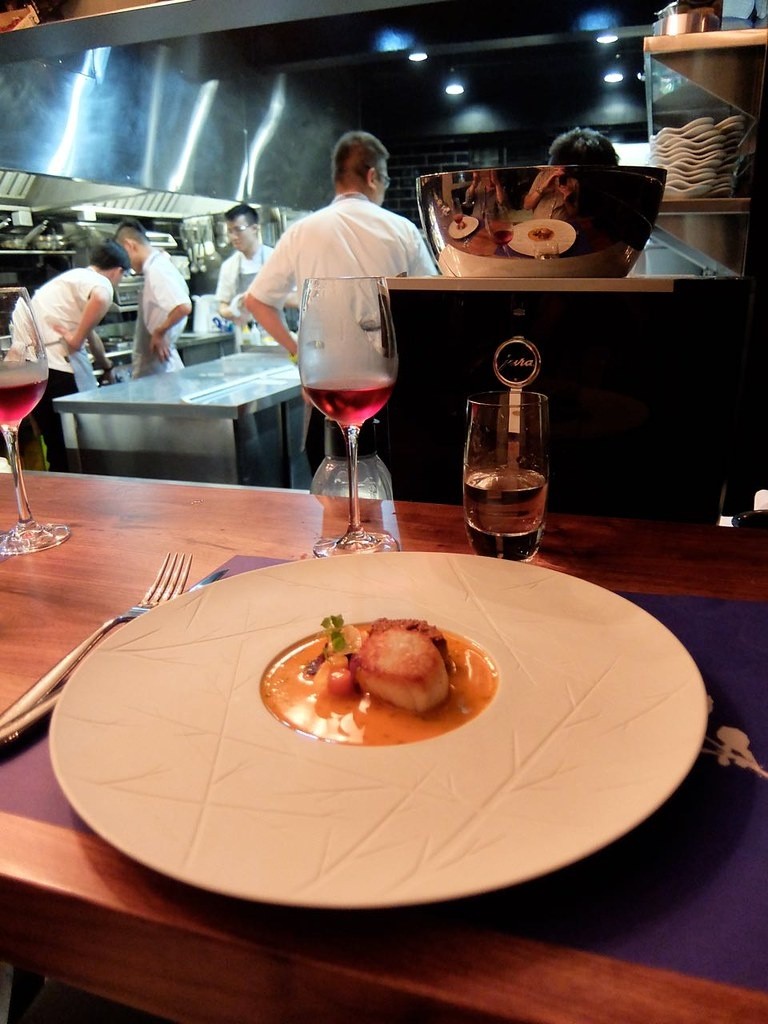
321, 614, 347, 660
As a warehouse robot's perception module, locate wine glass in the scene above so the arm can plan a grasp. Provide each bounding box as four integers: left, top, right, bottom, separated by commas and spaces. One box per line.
448, 197, 472, 248
0, 287, 71, 556
483, 205, 514, 257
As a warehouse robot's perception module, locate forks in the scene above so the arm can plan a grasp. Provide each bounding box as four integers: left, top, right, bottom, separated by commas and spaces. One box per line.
0, 551, 193, 717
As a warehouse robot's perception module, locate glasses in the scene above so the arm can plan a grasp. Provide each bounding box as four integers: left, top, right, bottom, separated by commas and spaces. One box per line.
364, 164, 391, 190
226, 222, 256, 237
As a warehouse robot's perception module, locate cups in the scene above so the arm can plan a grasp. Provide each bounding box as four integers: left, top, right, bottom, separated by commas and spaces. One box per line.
534, 241, 559, 260
462, 391, 550, 562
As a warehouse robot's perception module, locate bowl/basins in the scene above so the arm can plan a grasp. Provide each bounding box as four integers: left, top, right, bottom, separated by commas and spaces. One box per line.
414, 166, 669, 277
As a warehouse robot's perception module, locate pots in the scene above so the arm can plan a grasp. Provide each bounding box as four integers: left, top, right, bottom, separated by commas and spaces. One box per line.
0, 216, 89, 250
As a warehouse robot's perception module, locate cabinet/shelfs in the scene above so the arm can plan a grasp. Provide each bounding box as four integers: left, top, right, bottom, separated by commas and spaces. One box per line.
644, 28, 768, 217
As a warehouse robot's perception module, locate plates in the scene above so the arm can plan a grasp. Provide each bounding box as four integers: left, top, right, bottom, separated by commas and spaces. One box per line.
47, 551, 708, 910
448, 216, 479, 239
508, 219, 577, 256
642, 114, 747, 197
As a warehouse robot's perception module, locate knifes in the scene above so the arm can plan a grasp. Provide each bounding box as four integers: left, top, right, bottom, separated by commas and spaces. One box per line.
1, 568, 232, 742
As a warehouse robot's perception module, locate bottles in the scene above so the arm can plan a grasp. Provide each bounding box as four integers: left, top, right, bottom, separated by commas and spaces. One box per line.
239, 321, 262, 346
297, 274, 399, 559
308, 417, 392, 500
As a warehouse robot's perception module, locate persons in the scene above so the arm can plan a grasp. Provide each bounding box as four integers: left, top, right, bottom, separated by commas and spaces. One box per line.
523, 167, 581, 227
463, 170, 504, 221
112, 217, 192, 377
11, 242, 131, 473
217, 204, 300, 354
244, 130, 439, 479
550, 130, 647, 276
